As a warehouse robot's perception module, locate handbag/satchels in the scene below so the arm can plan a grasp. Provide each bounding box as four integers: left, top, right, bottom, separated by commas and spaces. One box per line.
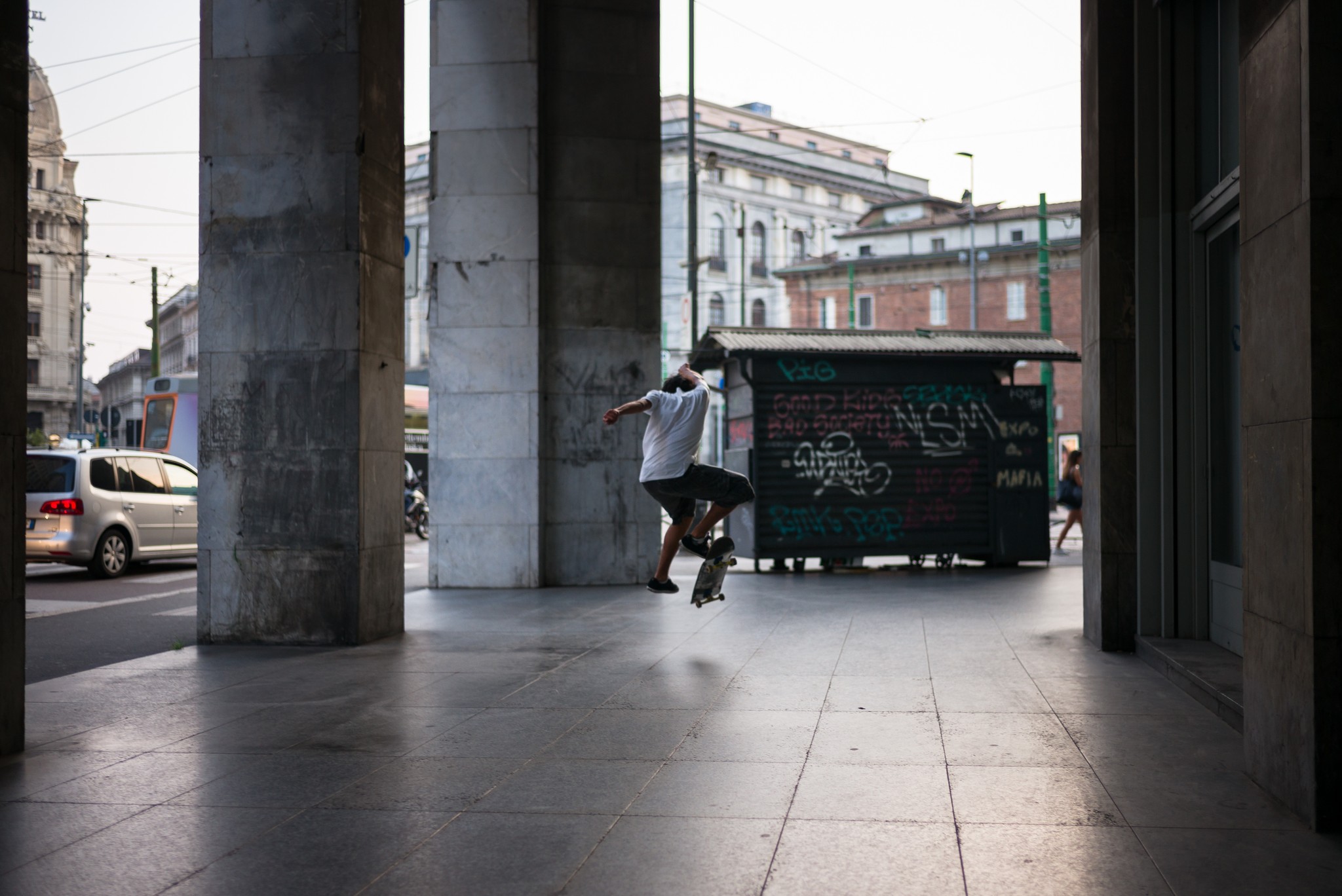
1057, 466, 1083, 511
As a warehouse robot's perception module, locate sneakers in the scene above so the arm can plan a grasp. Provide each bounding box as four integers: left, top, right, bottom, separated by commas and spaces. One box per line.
647, 575, 679, 593
678, 534, 711, 558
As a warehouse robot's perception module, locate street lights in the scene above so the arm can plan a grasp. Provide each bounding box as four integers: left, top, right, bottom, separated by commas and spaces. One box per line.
957, 147, 978, 332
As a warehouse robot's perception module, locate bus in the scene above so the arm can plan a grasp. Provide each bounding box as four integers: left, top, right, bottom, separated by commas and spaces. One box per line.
139, 373, 197, 470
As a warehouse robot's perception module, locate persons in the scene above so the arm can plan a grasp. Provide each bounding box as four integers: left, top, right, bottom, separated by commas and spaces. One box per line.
603, 363, 757, 593
49, 434, 60, 448
1053, 450, 1084, 557
403, 459, 425, 529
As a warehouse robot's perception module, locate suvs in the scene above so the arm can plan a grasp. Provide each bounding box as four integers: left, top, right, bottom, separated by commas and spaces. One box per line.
26, 448, 200, 581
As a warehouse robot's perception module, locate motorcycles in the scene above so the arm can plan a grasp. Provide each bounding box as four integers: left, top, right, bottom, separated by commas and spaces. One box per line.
403, 470, 431, 540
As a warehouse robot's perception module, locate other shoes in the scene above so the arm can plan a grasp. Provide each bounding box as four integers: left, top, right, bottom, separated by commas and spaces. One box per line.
1053, 548, 1067, 556
